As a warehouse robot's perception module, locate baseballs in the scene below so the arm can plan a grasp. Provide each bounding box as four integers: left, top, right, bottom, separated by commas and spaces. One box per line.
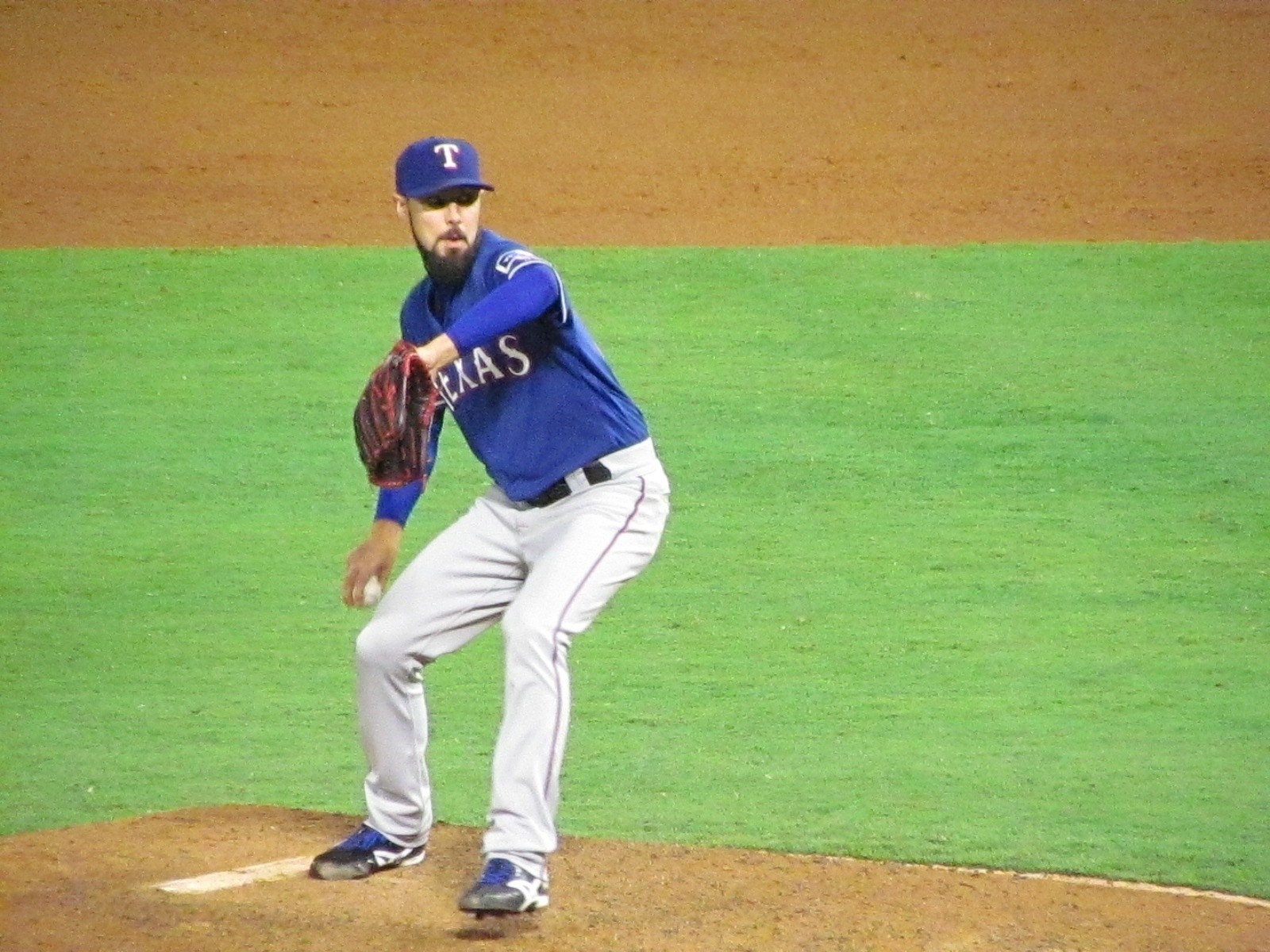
362, 576, 381, 606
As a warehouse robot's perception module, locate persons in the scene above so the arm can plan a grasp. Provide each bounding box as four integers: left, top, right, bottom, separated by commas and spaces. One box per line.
308, 135, 671, 912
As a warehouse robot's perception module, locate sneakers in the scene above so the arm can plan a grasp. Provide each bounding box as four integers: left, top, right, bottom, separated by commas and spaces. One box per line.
309, 825, 427, 881
458, 859, 550, 916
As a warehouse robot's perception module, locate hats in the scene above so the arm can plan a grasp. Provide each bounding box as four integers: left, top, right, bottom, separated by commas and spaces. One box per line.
395, 136, 494, 199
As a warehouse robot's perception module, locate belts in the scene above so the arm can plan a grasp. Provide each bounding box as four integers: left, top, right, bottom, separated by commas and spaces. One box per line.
522, 461, 612, 508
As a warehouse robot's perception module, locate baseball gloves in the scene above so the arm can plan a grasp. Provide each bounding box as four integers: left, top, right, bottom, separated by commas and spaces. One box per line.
352, 340, 435, 489
